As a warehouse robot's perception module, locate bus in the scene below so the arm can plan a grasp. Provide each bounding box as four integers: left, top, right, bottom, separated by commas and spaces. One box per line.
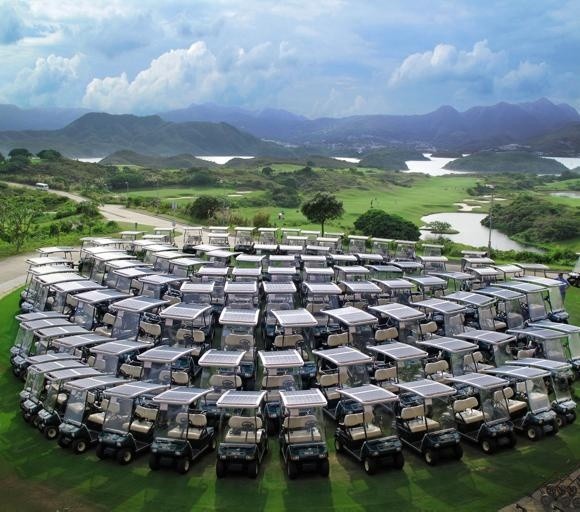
35, 182, 49, 192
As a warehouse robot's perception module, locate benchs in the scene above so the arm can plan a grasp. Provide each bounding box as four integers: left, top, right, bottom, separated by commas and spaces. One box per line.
19, 231, 579, 443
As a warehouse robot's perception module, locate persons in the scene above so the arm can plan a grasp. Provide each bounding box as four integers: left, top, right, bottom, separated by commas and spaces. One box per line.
555, 271, 571, 305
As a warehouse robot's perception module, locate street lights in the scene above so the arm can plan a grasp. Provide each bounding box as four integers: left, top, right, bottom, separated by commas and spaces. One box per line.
154, 176, 159, 214
485, 184, 495, 257
125, 180, 128, 204
216, 175, 225, 226
